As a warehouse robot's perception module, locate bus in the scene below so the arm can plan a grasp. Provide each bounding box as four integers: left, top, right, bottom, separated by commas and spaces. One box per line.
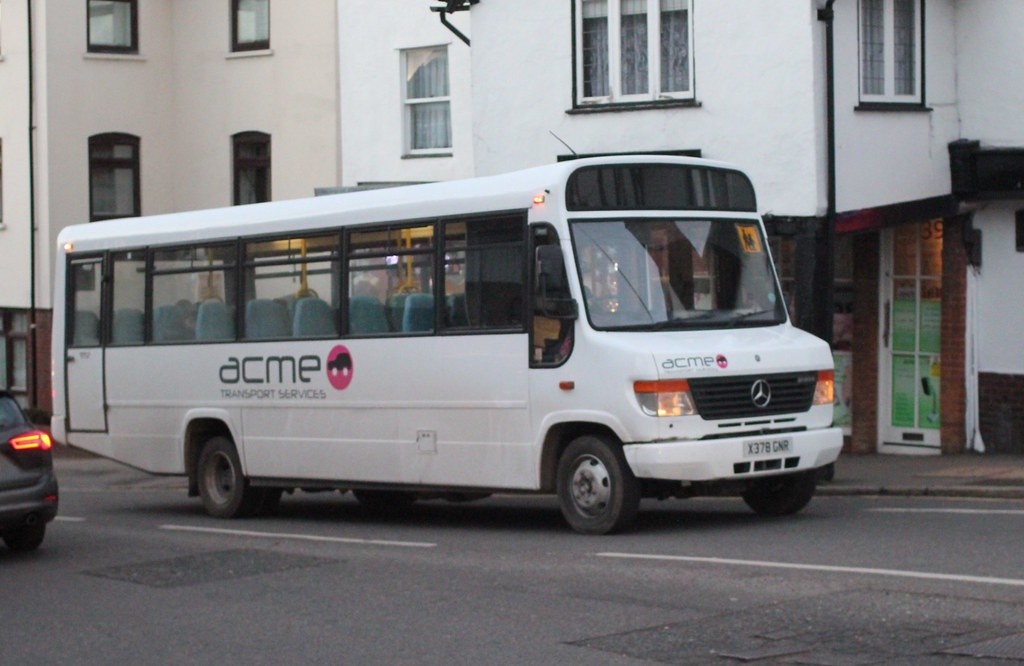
48, 152, 847, 540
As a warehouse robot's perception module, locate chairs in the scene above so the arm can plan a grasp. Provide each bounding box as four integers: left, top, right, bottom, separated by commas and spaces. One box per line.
114, 308, 146, 344
153, 306, 194, 341
75, 311, 99, 345
177, 298, 194, 322
348, 296, 389, 335
196, 299, 235, 340
247, 299, 290, 338
385, 296, 405, 330
292, 297, 337, 337
447, 293, 470, 327
400, 294, 434, 333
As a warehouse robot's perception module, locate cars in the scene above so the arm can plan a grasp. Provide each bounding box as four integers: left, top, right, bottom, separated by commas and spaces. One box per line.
0, 387, 59, 550
